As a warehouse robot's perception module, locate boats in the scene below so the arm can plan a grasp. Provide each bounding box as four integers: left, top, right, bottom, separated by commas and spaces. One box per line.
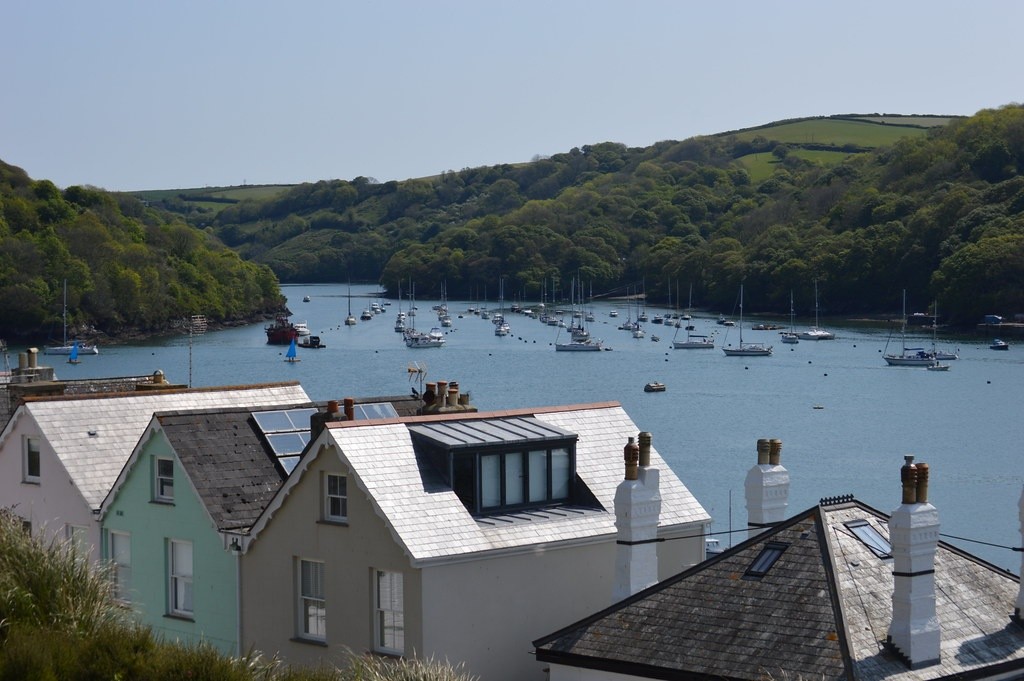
989, 339, 1009, 351
263, 308, 298, 345
303, 296, 310, 302
297, 335, 326, 349
294, 323, 310, 336
706, 539, 725, 559
928, 364, 950, 371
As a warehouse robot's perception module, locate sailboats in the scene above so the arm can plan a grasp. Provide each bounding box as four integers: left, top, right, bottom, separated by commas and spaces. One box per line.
42, 278, 99, 355
881, 288, 936, 365
283, 336, 301, 362
65, 342, 82, 365
917, 321, 958, 360
344, 275, 836, 356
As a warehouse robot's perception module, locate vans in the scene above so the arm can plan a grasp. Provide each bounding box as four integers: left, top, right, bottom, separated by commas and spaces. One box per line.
985, 315, 1006, 324
